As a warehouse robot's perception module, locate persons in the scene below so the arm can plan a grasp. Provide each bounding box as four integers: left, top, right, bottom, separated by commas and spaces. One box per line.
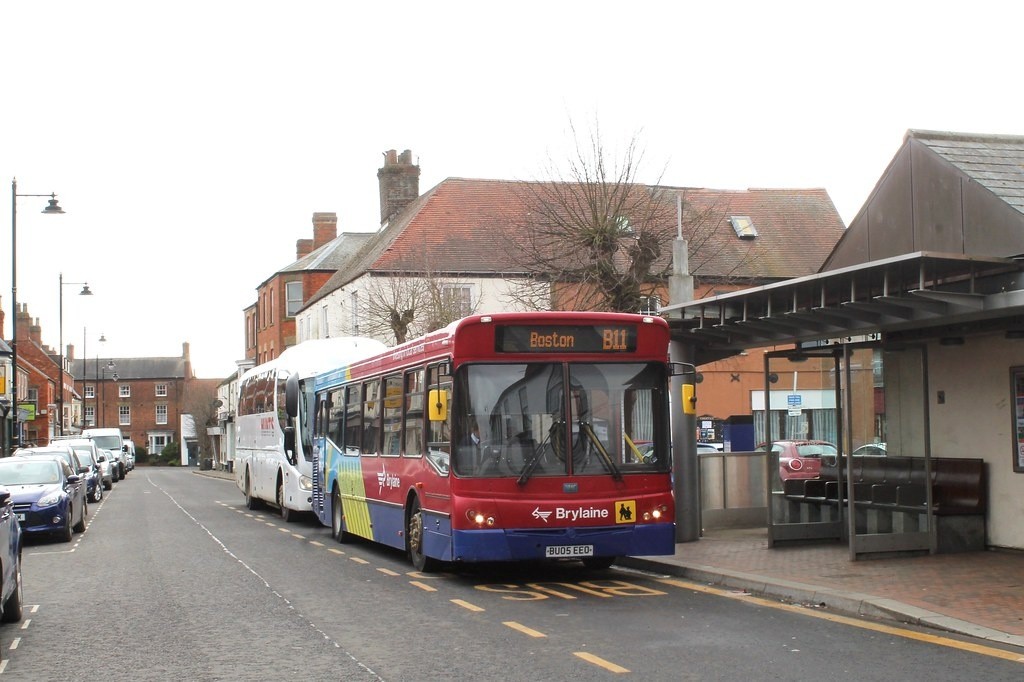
459, 422, 487, 449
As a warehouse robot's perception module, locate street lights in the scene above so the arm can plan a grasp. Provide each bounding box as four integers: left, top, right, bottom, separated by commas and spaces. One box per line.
57, 273, 96, 436
81, 324, 120, 429
11, 177, 67, 453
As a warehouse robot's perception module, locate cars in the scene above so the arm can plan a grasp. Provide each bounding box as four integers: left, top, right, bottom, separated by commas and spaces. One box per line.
846, 443, 890, 458
0, 490, 23, 625
0, 428, 136, 541
639, 440, 719, 466
756, 439, 837, 480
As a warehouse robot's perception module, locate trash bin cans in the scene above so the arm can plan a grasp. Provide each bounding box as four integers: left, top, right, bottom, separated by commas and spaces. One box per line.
199, 458, 213, 470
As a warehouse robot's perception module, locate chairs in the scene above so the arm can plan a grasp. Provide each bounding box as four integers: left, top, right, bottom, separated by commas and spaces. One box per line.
782, 453, 986, 551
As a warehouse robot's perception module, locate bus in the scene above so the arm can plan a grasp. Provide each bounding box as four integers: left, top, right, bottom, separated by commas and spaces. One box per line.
305, 312, 697, 572
236, 335, 391, 523
